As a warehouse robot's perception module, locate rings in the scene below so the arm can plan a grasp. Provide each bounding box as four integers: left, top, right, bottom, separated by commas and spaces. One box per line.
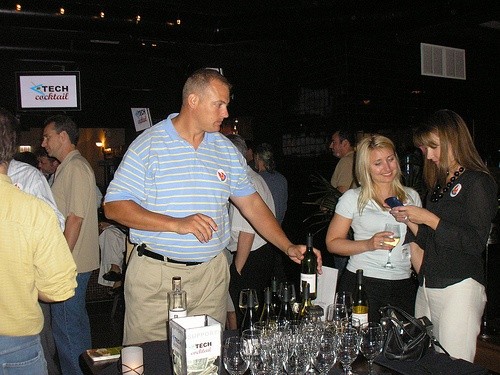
404, 216, 408, 219
380, 242, 383, 246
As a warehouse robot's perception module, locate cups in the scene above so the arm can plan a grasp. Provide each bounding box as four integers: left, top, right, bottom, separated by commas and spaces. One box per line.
239, 289, 259, 316
122, 346, 144, 375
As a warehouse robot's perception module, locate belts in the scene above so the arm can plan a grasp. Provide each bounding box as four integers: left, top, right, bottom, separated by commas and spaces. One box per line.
137, 246, 217, 266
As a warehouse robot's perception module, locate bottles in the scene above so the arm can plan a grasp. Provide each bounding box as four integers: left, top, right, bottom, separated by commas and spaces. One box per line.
279, 288, 297, 335
268, 275, 281, 317
296, 282, 318, 325
300, 232, 318, 301
350, 269, 369, 330
167, 277, 187, 325
240, 290, 259, 335
258, 287, 279, 327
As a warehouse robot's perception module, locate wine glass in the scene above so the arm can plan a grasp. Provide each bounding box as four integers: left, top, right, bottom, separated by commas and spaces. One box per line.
359, 321, 385, 375
382, 223, 400, 270
223, 291, 362, 375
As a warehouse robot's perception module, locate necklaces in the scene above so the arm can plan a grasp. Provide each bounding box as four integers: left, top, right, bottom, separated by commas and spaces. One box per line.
430, 166, 465, 201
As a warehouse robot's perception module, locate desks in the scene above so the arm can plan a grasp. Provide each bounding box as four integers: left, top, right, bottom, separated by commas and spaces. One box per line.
80, 318, 500, 375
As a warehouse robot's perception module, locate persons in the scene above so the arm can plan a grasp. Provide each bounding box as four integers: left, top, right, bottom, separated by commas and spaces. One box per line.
383, 108, 500, 363
225, 134, 276, 326
103, 69, 322, 345
246, 146, 259, 173
321, 129, 356, 274
254, 143, 288, 280
326, 135, 422, 321
98, 225, 132, 293
41, 115, 99, 375
0, 119, 78, 375
